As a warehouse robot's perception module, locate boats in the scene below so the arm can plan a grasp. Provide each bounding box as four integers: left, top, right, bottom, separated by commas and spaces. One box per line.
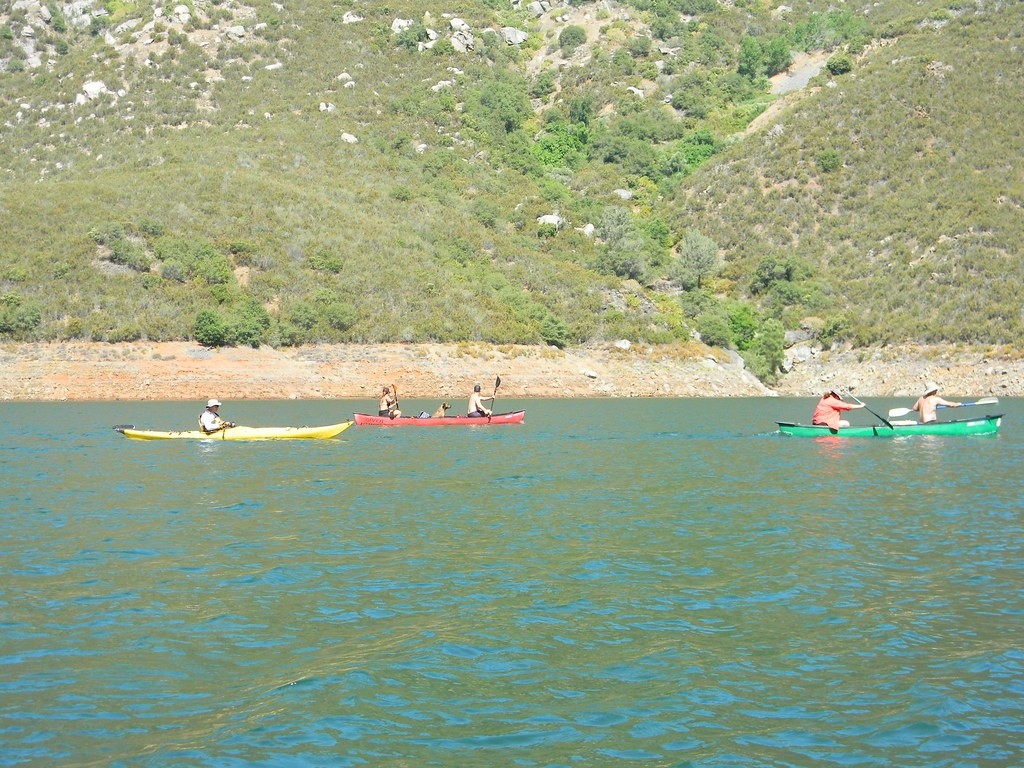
353, 409, 527, 426
113, 417, 354, 442
774, 413, 1007, 438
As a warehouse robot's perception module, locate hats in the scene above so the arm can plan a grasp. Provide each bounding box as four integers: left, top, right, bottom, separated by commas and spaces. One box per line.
921, 386, 939, 397
831, 388, 844, 400
474, 385, 480, 392
383, 388, 391, 393
205, 399, 222, 408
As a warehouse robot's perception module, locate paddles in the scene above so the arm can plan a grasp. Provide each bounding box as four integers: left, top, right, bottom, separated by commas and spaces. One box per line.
843, 389, 894, 430
392, 383, 400, 410
491, 375, 501, 411
204, 421, 235, 432
888, 396, 1000, 417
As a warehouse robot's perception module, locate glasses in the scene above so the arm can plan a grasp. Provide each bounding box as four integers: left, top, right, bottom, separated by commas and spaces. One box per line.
213, 404, 220, 407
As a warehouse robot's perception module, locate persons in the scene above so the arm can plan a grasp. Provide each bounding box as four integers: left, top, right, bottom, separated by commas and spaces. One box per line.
813, 389, 866, 432
200, 399, 236, 431
379, 387, 401, 419
468, 385, 495, 417
915, 380, 962, 423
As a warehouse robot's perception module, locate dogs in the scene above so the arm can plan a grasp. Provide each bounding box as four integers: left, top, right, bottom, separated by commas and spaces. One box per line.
431, 402, 451, 417
